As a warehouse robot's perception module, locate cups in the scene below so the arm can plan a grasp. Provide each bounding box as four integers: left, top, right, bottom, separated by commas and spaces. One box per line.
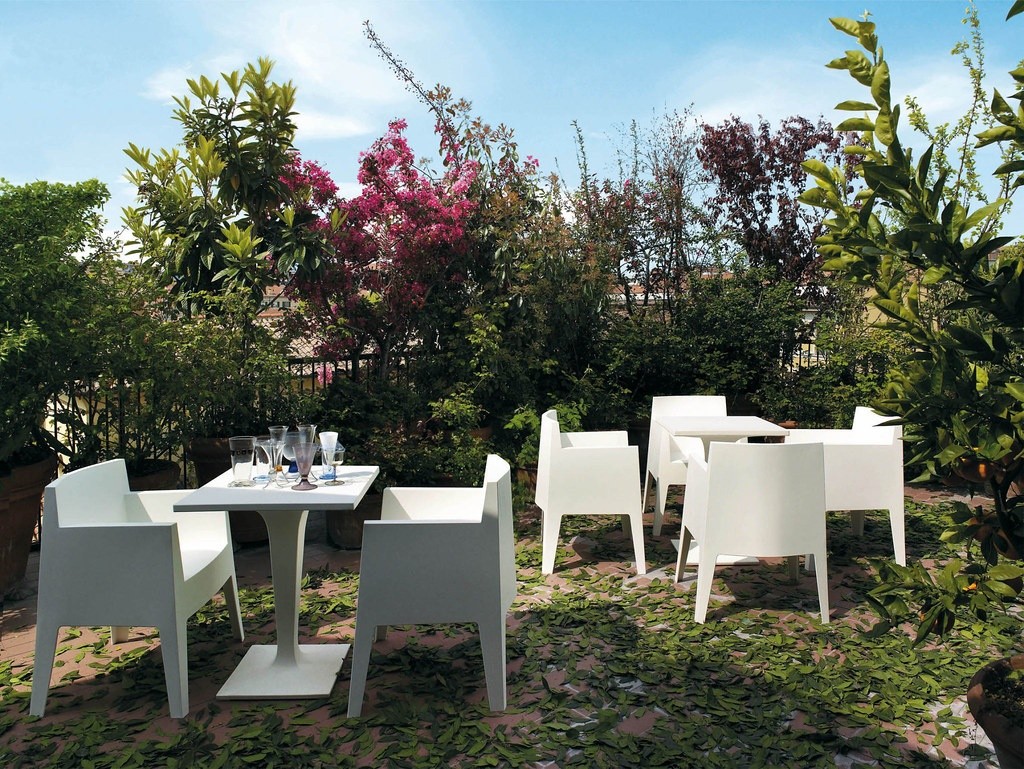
253, 424, 317, 491
229, 436, 256, 481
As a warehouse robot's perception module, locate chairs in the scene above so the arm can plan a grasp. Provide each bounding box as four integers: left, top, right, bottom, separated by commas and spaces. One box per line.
642, 395, 748, 536
29, 458, 244, 718
675, 442, 829, 624
535, 410, 647, 575
785, 406, 906, 571
348, 454, 516, 717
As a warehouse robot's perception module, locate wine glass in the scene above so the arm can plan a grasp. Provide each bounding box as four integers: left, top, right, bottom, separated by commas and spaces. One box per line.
324, 448, 345, 485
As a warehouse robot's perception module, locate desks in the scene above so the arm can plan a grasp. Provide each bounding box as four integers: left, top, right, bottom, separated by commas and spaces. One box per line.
173, 465, 379, 701
651, 415, 789, 565
